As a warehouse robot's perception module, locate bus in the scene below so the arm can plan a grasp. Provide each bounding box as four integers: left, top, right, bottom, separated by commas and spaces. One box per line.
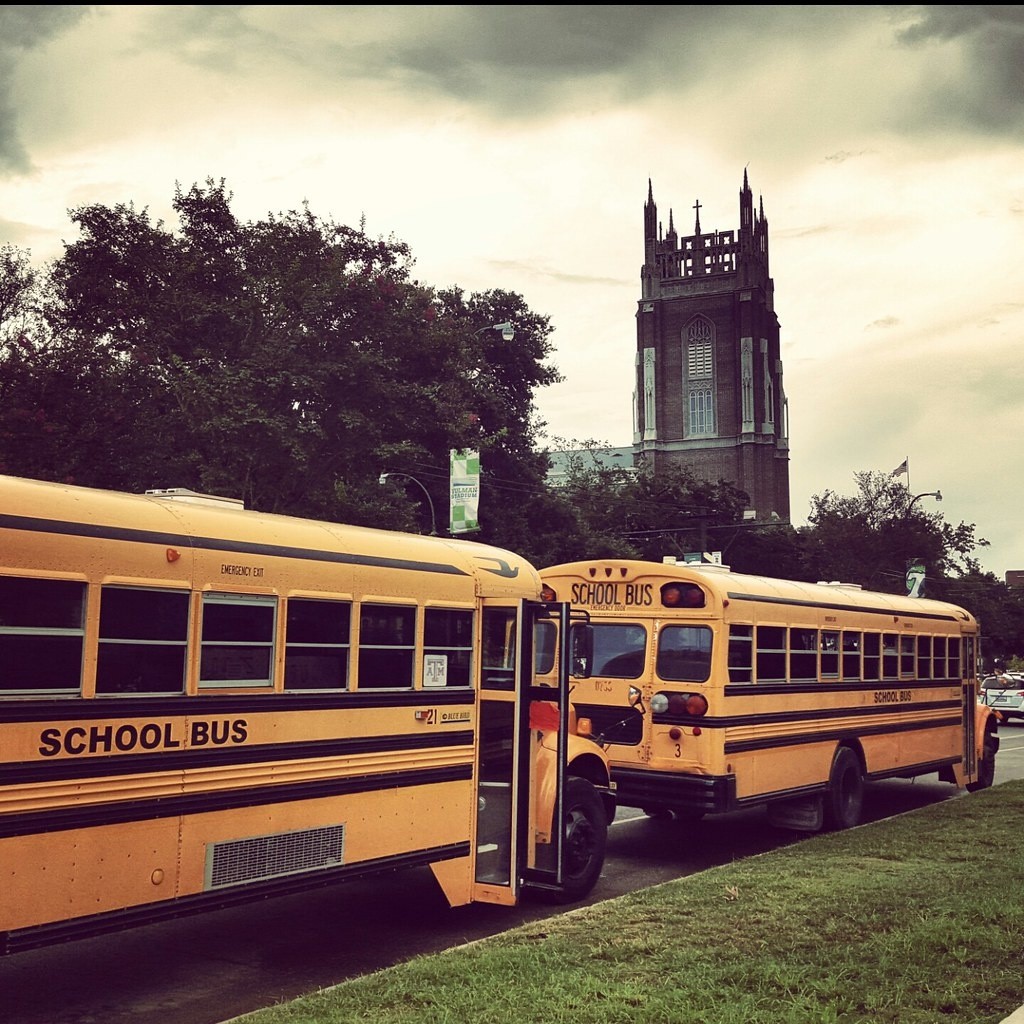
1, 471, 647, 958
503, 559, 1017, 836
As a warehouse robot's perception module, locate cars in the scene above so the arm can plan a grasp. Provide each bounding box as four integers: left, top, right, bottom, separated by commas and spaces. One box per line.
975, 667, 1024, 723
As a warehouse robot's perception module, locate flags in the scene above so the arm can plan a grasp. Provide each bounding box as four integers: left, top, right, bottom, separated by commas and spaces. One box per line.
892, 461, 907, 477
